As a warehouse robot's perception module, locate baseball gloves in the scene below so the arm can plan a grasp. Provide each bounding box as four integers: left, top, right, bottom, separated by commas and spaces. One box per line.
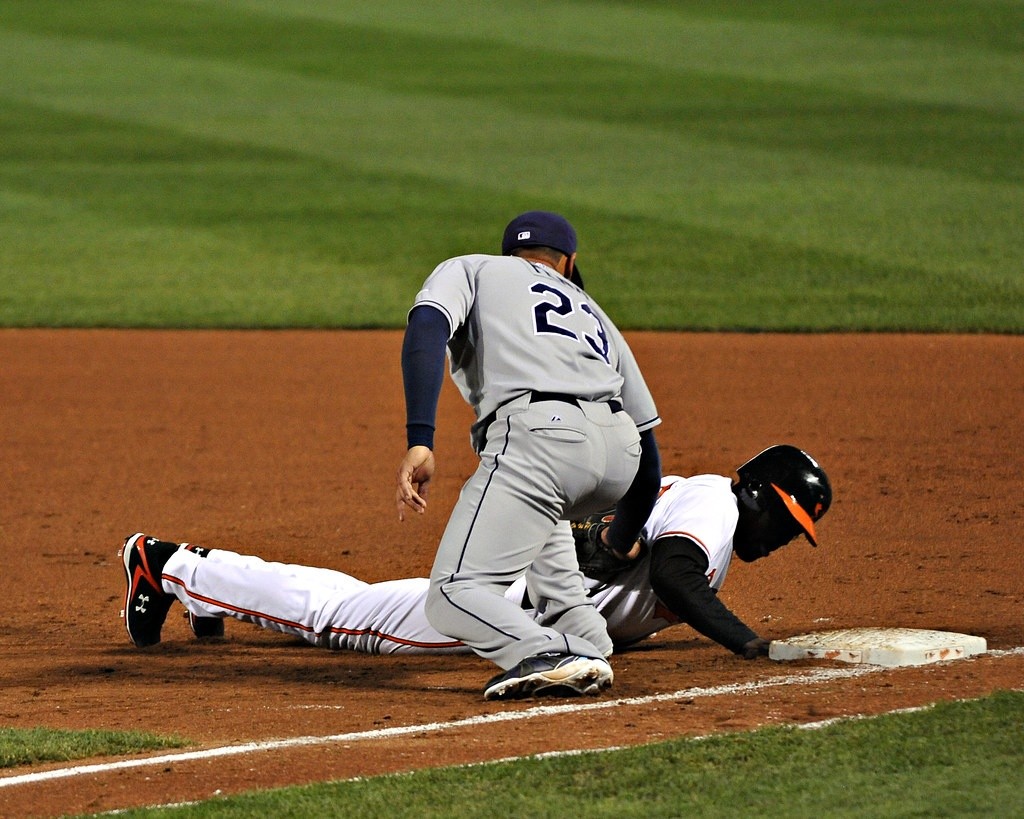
570, 506, 652, 587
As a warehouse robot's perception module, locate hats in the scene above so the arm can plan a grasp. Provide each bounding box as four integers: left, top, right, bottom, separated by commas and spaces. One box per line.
502, 210, 585, 290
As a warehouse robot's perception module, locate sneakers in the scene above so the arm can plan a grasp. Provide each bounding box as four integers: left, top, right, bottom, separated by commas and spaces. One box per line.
183, 605, 224, 639
484, 650, 614, 699
122, 532, 178, 645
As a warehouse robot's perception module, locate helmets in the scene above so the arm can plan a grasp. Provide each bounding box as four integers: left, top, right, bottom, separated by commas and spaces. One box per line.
732, 445, 831, 548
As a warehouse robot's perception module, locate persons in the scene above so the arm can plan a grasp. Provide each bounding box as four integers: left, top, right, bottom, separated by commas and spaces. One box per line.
397, 211, 662, 701
123, 443, 832, 656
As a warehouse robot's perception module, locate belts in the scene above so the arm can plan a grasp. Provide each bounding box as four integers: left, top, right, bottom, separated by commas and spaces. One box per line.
484, 391, 623, 425
